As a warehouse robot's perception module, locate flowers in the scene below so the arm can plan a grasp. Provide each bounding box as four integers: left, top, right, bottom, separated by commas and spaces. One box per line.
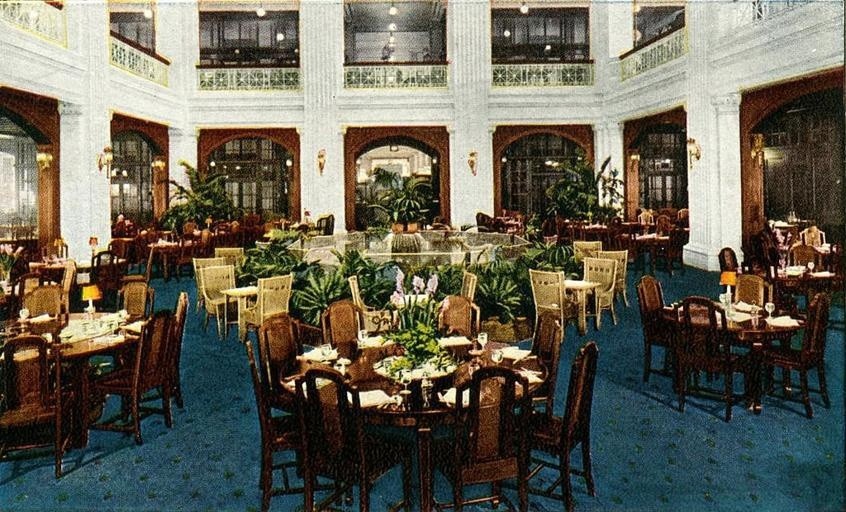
303, 208, 310, 215
0, 244, 24, 291
388, 268, 450, 378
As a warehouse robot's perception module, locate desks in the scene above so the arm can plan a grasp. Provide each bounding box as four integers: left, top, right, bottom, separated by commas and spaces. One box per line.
583, 225, 609, 251
636, 233, 665, 274
773, 274, 832, 302
148, 241, 178, 282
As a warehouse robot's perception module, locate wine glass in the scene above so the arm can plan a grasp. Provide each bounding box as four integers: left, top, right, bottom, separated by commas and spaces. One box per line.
399, 370, 412, 394
719, 294, 730, 314
20, 309, 28, 321
492, 352, 503, 367
334, 364, 346, 379
358, 330, 367, 344
808, 262, 815, 274
42, 332, 53, 354
321, 344, 333, 361
477, 332, 487, 352
751, 305, 758, 326
765, 303, 775, 318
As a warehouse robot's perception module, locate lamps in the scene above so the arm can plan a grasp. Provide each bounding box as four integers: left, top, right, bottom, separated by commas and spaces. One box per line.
389, 1, 397, 15
505, 28, 513, 38
81, 285, 100, 314
210, 158, 218, 173
36, 152, 52, 172
317, 150, 327, 175
86, 237, 100, 262
751, 146, 765, 172
276, 30, 285, 42
141, 3, 152, 20
629, 152, 640, 174
256, 1, 267, 17
686, 136, 699, 163
52, 238, 63, 265
520, 3, 529, 17
467, 152, 481, 177
205, 218, 212, 231
282, 155, 291, 172
719, 271, 739, 318
386, 38, 396, 52
99, 147, 115, 174
389, 22, 399, 33
152, 158, 165, 183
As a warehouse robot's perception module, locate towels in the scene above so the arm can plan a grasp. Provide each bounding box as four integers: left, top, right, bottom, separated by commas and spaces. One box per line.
769, 315, 799, 328
734, 301, 760, 312
518, 371, 541, 384
363, 336, 382, 349
439, 336, 470, 349
286, 378, 331, 393
297, 342, 337, 362
440, 388, 484, 405
346, 389, 397, 409
123, 323, 143, 333
502, 348, 530, 360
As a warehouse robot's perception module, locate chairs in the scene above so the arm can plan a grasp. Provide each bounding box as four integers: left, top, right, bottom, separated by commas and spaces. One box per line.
718, 248, 739, 292
678, 208, 688, 223
512, 311, 560, 416
321, 302, 366, 343
349, 277, 397, 331
317, 213, 334, 235
192, 257, 227, 315
243, 214, 261, 242
652, 216, 670, 231
111, 239, 130, 259
477, 212, 494, 231
638, 211, 653, 224
296, 224, 308, 235
214, 247, 246, 264
256, 314, 303, 386
656, 227, 685, 273
636, 275, 684, 384
120, 290, 189, 409
88, 310, 171, 446
573, 241, 602, 265
439, 293, 474, 335
244, 342, 344, 512
22, 285, 66, 323
121, 246, 152, 282
785, 245, 822, 305
12, 273, 51, 321
440, 271, 481, 331
495, 216, 522, 236
800, 226, 826, 248
747, 293, 831, 418
200, 264, 237, 339
814, 244, 840, 293
732, 274, 772, 309
183, 223, 198, 235
212, 224, 234, 250
130, 236, 146, 274
0, 336, 73, 478
117, 280, 153, 321
781, 227, 798, 247
227, 221, 241, 245
597, 250, 630, 307
672, 296, 748, 421
273, 220, 291, 232
609, 223, 638, 262
55, 261, 76, 312
240, 275, 292, 340
582, 258, 617, 327
176, 235, 199, 283
503, 209, 519, 217
199, 229, 214, 256
145, 231, 158, 243
527, 269, 575, 343
294, 366, 412, 512
494, 341, 599, 512
430, 365, 529, 512
90, 252, 123, 297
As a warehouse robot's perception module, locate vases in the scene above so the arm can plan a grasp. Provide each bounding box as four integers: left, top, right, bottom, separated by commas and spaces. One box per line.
303, 216, 309, 224
